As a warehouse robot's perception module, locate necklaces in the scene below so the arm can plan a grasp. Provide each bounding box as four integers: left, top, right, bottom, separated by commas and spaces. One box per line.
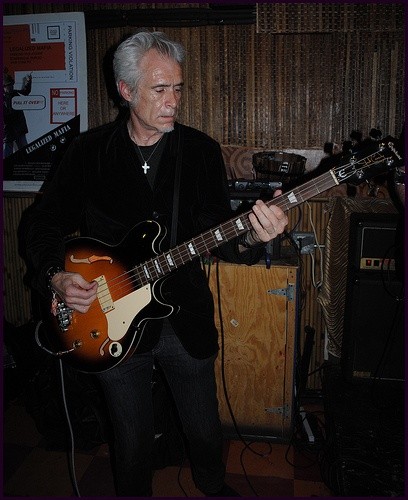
129, 118, 163, 174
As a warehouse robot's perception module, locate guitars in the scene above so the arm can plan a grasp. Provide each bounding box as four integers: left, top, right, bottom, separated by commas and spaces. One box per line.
31, 141, 407, 374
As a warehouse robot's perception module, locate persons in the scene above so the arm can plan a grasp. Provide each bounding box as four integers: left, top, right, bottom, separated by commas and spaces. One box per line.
25, 31, 289, 498
3, 73, 33, 158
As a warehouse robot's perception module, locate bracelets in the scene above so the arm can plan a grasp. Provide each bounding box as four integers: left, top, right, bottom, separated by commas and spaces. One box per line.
239, 232, 261, 248
47, 266, 65, 316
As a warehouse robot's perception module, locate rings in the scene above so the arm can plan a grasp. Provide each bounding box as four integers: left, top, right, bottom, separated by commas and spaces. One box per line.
267, 229, 275, 234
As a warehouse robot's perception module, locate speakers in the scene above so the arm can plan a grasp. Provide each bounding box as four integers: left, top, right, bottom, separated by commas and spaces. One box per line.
320, 370, 406, 497
343, 213, 408, 379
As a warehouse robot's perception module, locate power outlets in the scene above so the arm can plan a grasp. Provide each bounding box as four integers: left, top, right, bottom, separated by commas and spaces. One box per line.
294, 232, 317, 254
